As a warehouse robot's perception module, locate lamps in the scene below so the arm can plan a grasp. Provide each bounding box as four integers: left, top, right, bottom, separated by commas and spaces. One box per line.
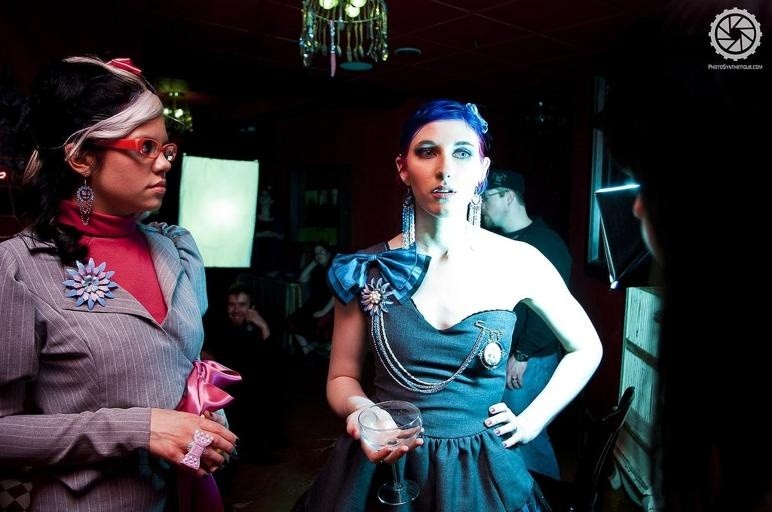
298, 1, 389, 67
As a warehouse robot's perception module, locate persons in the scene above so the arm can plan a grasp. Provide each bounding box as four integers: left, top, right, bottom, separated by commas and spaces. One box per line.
0, 57, 240, 512
291, 100, 603, 512
227, 282, 297, 369
298, 239, 334, 343
480, 168, 573, 481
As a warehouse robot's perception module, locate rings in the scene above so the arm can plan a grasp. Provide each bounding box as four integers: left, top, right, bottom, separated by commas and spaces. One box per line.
181, 428, 214, 470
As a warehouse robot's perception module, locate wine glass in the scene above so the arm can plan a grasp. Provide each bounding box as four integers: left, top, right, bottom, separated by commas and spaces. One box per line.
358, 400, 422, 506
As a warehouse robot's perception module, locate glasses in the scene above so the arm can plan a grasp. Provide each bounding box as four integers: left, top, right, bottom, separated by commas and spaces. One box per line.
92, 137, 178, 162
